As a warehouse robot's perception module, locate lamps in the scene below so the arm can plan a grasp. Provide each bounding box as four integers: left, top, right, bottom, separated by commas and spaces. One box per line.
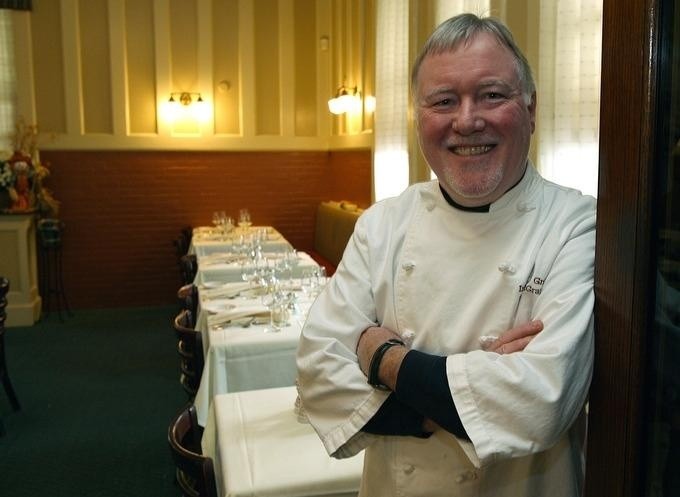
159, 92, 211, 122
328, 86, 360, 114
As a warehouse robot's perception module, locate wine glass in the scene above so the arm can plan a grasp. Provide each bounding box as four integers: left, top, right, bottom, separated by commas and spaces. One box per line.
212, 209, 329, 330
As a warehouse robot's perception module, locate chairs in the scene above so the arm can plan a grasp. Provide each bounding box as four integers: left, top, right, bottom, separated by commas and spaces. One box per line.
167, 255, 219, 497
0, 278, 21, 438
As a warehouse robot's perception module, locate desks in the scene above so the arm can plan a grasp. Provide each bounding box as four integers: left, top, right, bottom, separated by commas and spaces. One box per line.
190, 225, 364, 497
313, 200, 370, 271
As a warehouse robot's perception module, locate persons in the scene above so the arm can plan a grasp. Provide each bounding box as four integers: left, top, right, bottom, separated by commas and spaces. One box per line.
291, 10, 597, 496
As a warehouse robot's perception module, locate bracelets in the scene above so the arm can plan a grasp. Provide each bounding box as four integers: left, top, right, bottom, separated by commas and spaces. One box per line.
365, 335, 405, 391
